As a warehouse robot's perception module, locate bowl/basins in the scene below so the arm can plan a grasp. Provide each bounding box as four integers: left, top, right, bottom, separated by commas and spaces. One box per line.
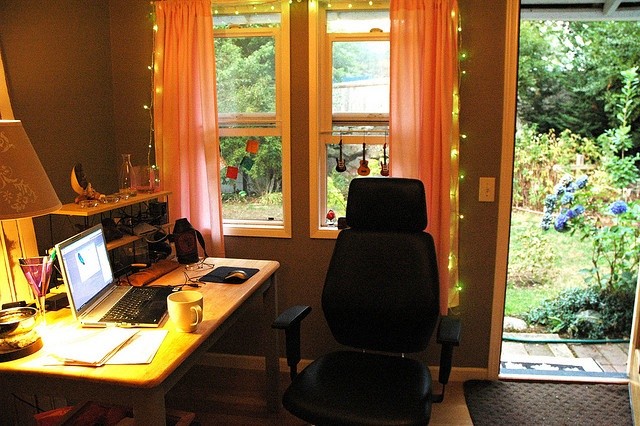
0, 305, 40, 337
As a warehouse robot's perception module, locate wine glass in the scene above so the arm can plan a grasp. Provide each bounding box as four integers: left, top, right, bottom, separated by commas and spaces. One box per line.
19, 257, 56, 324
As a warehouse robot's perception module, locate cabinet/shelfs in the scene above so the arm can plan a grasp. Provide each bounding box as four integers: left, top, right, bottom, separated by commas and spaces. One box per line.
50, 190, 174, 281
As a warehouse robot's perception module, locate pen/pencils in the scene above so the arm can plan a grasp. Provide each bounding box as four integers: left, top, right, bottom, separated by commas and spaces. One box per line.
38, 250, 56, 288
41, 256, 48, 315
19, 258, 40, 294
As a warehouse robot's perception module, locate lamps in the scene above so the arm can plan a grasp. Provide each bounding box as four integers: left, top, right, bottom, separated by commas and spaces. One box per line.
0, 119, 63, 307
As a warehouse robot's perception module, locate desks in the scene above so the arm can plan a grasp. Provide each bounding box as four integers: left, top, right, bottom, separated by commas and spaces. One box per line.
0, 250, 280, 426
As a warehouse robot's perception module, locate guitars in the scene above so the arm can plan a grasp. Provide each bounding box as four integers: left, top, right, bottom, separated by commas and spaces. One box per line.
380, 143, 389, 176
335, 140, 346, 172
357, 142, 370, 176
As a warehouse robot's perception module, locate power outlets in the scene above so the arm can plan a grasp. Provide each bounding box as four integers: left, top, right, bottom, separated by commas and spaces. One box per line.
479, 177, 496, 202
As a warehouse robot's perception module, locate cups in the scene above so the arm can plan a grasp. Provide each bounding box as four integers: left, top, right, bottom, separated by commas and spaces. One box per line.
165, 292, 204, 333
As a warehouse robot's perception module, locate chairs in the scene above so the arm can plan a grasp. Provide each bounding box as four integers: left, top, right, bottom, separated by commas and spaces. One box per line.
271, 178, 462, 426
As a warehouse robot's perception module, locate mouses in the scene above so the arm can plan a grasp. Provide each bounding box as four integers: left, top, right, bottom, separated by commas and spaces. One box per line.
224, 269, 247, 282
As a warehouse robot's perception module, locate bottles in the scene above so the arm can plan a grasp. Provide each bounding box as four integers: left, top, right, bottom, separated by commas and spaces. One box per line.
173, 217, 199, 263
119, 153, 137, 196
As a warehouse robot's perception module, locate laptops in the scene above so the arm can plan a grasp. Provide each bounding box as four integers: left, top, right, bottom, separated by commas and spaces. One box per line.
55, 223, 182, 328
